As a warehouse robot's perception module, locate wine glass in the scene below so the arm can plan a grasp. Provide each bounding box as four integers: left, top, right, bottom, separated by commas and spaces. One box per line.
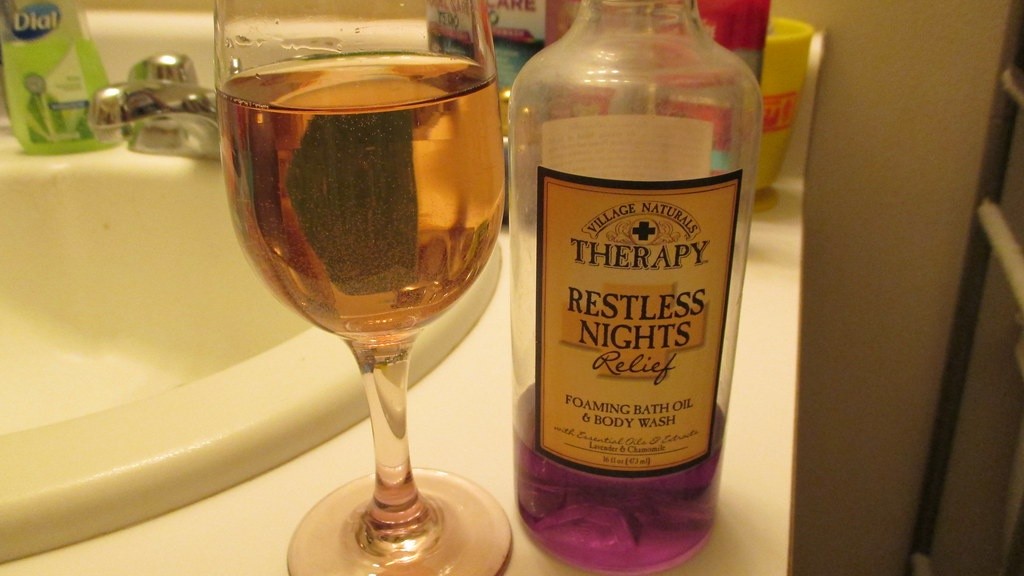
212, 0, 514, 576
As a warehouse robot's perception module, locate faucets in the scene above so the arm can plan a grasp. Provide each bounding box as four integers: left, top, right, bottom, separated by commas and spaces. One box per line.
84, 49, 221, 161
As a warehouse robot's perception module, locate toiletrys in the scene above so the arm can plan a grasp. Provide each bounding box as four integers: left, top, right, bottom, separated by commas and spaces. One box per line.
0, 0, 120, 154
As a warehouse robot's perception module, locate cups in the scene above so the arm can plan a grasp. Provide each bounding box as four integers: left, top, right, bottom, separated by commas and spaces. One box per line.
756, 15, 815, 187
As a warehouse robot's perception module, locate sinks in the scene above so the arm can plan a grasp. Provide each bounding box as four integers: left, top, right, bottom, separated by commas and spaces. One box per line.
0, 119, 510, 563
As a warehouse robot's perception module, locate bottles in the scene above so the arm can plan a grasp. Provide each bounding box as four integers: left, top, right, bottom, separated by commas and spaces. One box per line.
505, 1, 763, 576
